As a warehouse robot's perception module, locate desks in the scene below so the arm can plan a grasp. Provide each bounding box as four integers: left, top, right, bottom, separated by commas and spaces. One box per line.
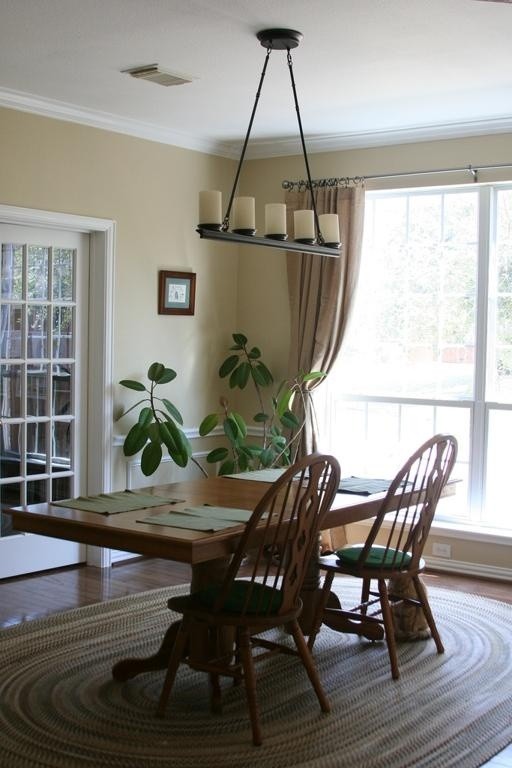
2, 463, 471, 720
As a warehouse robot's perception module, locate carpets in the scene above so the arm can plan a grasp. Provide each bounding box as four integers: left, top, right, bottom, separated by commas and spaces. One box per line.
1, 571, 512, 767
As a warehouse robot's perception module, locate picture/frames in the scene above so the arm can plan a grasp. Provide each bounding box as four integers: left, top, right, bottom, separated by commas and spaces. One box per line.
158, 269, 197, 316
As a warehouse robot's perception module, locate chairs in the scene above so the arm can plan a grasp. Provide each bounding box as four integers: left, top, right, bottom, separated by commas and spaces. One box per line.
310, 433, 459, 686
153, 452, 343, 746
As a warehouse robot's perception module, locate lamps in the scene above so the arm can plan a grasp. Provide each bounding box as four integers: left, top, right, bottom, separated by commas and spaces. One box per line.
195, 26, 345, 261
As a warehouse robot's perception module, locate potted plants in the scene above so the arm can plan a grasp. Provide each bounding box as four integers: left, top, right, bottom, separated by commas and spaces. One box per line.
114, 329, 327, 569
